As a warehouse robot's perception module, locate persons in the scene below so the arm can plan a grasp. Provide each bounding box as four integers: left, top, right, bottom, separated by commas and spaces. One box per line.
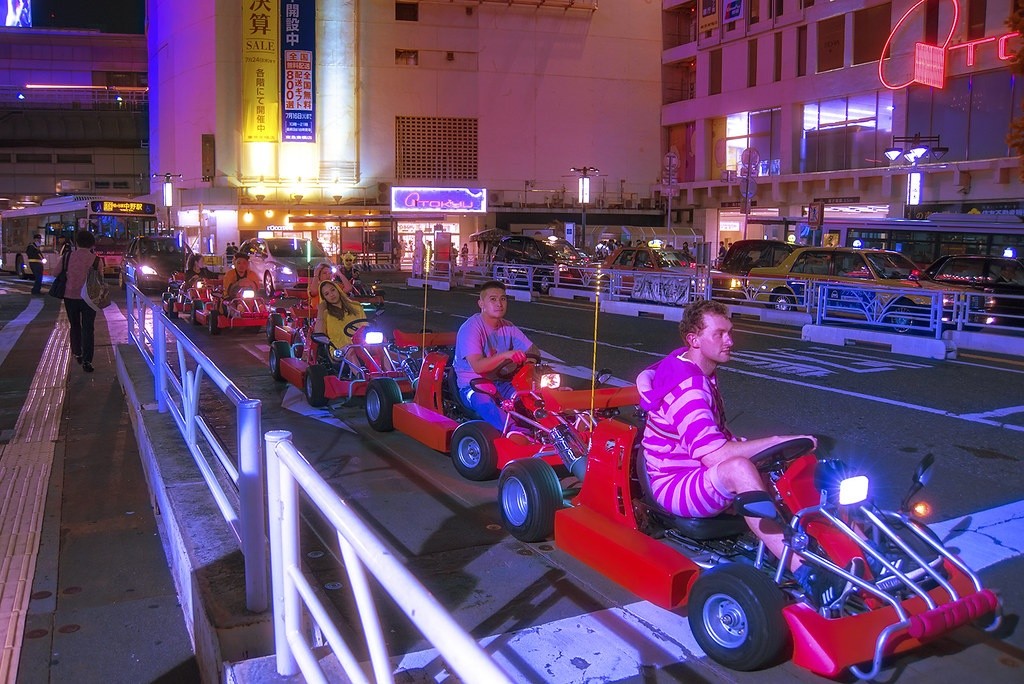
683, 242, 697, 257
996, 263, 1015, 283
594, 239, 618, 260
452, 281, 590, 447
307, 253, 392, 379
901, 233, 916, 260
166, 244, 177, 254
184, 254, 225, 289
838, 258, 865, 278
223, 251, 260, 316
718, 240, 733, 269
635, 299, 943, 616
451, 243, 458, 266
460, 243, 468, 266
25, 234, 45, 297
53, 230, 104, 373
225, 241, 238, 266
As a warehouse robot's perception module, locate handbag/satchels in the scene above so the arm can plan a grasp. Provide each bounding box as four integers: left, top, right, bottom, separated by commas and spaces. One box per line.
49, 270, 66, 298
80, 267, 111, 312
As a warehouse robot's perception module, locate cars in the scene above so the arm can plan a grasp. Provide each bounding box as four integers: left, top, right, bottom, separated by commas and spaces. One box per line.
117, 235, 197, 295
232, 236, 340, 297
491, 234, 598, 295
722, 239, 807, 280
744, 246, 987, 334
923, 253, 1024, 332
581, 245, 746, 310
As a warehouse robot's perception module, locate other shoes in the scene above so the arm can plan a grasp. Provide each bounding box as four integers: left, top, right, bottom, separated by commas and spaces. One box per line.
236, 304, 244, 312
83, 363, 94, 372
258, 304, 264, 312
575, 411, 590, 432
30, 288, 44, 297
506, 431, 540, 446
77, 357, 82, 364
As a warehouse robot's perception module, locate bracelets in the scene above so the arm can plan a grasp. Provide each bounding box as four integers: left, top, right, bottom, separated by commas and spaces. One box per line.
316, 318, 324, 320
312, 276, 319, 277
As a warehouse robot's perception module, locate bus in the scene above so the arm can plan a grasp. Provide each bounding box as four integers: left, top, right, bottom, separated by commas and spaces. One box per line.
745, 210, 1024, 268
0, 193, 158, 282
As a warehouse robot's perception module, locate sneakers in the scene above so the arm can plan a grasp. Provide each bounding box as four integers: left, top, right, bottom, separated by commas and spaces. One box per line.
874, 554, 944, 592
819, 556, 865, 609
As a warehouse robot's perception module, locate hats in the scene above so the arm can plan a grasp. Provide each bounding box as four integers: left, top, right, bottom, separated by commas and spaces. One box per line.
34, 234, 41, 239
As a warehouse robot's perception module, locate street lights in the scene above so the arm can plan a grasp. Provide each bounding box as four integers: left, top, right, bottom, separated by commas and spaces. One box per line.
152, 173, 184, 235
569, 165, 600, 253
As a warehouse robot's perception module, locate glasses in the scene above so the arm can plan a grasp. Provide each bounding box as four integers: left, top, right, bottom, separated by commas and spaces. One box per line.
345, 259, 352, 262
